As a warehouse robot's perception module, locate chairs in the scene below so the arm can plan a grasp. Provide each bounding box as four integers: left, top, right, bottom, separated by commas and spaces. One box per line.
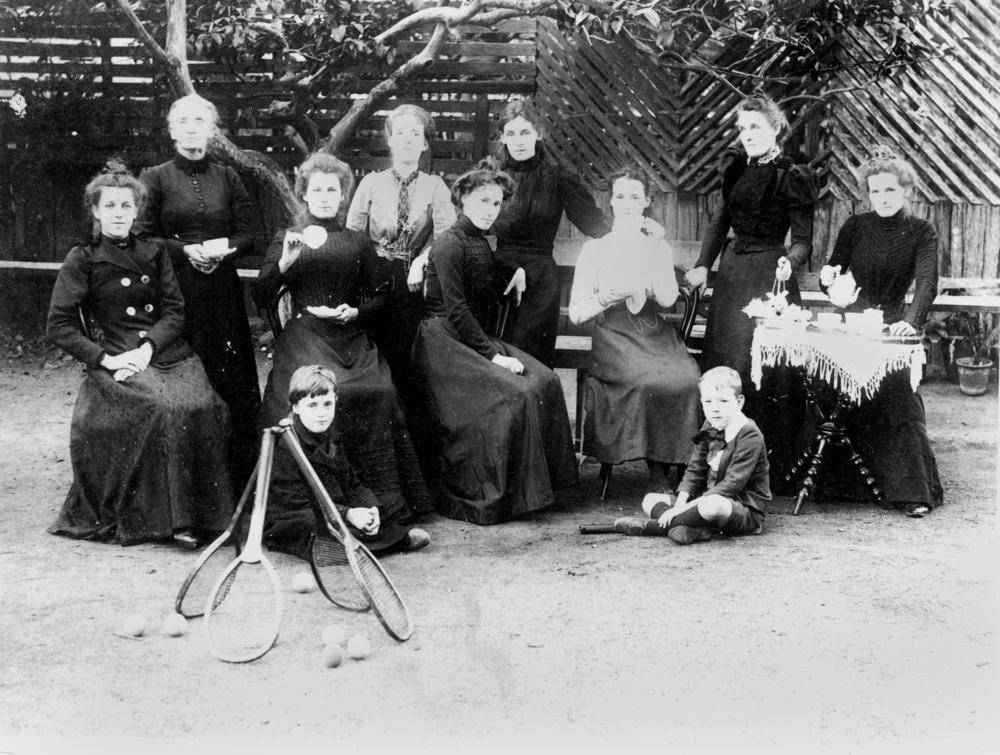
597, 265, 699, 500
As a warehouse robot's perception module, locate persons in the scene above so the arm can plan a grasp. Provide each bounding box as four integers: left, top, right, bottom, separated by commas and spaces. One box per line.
131, 95, 292, 515
47, 158, 242, 549
684, 89, 816, 495
819, 145, 944, 515
251, 153, 433, 521
568, 162, 701, 497
411, 154, 581, 526
346, 104, 461, 372
240, 364, 431, 562
488, 100, 666, 371
641, 366, 771, 544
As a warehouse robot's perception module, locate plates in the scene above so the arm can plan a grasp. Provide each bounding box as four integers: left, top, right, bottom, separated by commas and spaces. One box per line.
307, 307, 341, 317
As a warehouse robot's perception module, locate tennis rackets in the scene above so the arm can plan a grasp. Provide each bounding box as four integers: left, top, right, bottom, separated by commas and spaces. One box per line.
204, 424, 280, 668
172, 423, 285, 620
275, 416, 374, 615
275, 420, 416, 640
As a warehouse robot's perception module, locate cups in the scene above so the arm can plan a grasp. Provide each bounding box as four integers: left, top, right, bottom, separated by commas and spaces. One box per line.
818, 312, 842, 330
844, 308, 883, 336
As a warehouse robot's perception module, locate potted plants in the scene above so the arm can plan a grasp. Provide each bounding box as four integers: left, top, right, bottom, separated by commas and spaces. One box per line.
949, 316, 1000, 395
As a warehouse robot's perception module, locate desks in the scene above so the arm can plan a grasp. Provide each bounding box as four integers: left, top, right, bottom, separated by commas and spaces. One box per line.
750, 325, 924, 514
798, 290, 1000, 315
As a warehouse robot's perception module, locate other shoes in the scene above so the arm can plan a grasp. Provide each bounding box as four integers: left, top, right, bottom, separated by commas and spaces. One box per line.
175, 529, 197, 550
408, 528, 431, 550
614, 517, 656, 535
669, 525, 710, 544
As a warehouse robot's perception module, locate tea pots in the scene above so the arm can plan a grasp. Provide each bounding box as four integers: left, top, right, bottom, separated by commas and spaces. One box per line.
826, 268, 862, 307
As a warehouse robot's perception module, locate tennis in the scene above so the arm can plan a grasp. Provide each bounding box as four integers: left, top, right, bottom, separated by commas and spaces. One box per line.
292, 570, 312, 595
117, 613, 147, 638
320, 644, 342, 667
345, 634, 369, 660
163, 610, 185, 637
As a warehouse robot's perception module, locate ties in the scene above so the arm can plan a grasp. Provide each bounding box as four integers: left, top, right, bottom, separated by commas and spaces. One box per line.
392, 168, 420, 242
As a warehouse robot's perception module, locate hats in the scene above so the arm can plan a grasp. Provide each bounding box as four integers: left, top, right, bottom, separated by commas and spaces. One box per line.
450, 158, 515, 208
